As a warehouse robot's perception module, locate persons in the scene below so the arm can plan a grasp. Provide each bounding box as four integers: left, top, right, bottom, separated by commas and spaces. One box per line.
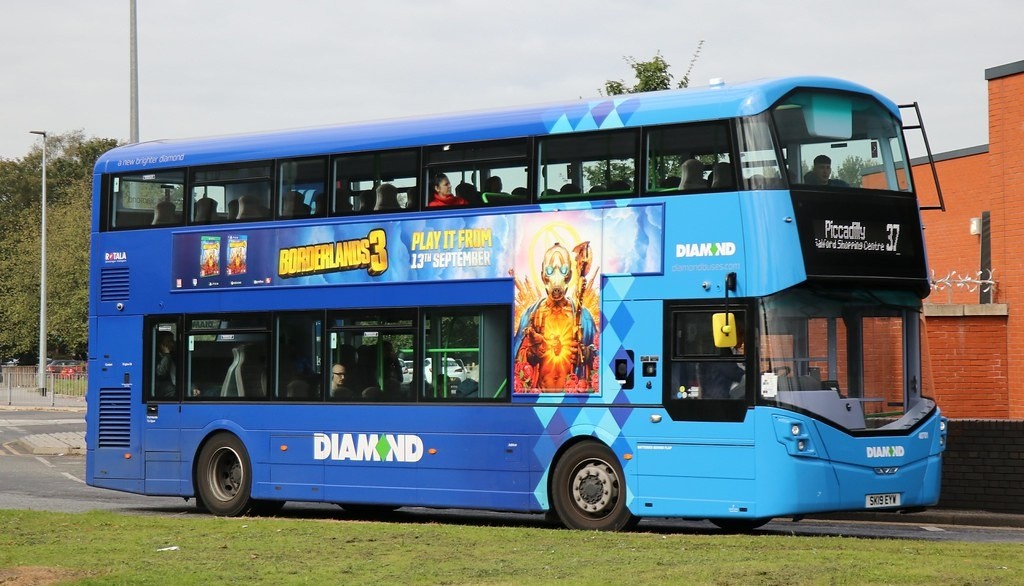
327, 364, 345, 397
426, 374, 452, 401
142, 331, 201, 400
805, 155, 834, 186
702, 338, 746, 400
427, 173, 469, 207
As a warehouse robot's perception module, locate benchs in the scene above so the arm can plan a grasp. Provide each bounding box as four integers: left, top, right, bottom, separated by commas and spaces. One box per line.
171, 344, 235, 394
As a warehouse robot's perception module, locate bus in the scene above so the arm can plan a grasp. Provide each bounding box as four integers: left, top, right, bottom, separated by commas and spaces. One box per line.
83, 74, 948, 534
397, 347, 480, 368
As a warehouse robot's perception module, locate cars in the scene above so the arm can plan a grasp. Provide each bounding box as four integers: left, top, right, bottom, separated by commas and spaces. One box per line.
47, 359, 88, 379
425, 358, 468, 387
397, 357, 414, 383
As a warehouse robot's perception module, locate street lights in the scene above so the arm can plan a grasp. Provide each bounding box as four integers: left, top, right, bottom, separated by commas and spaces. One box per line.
30, 130, 47, 395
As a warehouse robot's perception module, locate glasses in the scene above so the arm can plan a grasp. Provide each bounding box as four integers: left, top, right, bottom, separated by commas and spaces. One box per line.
333, 373, 345, 376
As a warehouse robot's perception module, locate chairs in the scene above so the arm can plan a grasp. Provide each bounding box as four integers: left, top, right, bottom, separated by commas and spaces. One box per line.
678, 159, 736, 191
149, 184, 425, 228
217, 343, 270, 399
